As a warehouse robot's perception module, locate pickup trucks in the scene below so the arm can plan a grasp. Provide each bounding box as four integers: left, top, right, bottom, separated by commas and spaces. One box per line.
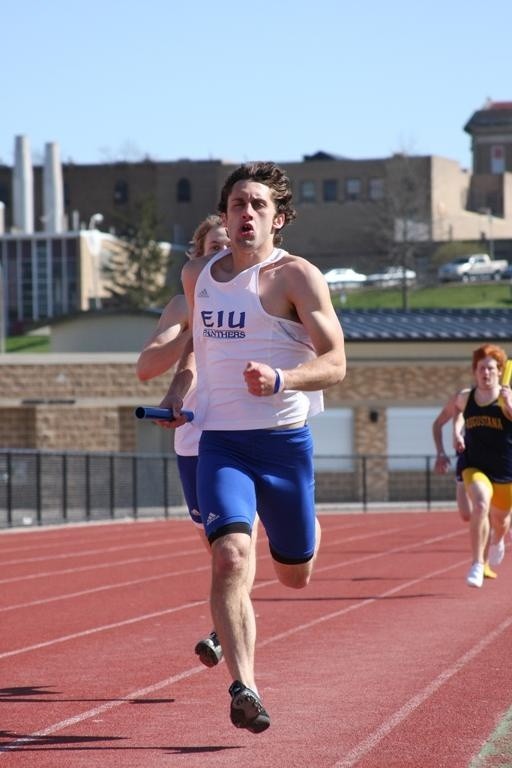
438, 253, 509, 283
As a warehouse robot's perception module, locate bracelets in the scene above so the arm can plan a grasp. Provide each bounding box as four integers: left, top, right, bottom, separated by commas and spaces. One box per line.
273, 367, 284, 393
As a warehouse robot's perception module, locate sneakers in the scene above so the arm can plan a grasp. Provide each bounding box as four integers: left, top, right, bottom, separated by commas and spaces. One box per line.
194, 630, 227, 666
228, 680, 271, 734
467, 530, 506, 587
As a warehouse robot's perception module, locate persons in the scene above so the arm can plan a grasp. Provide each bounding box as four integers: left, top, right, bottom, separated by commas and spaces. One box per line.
432, 382, 512, 579
454, 344, 512, 588
137, 215, 259, 667
153, 160, 348, 731
